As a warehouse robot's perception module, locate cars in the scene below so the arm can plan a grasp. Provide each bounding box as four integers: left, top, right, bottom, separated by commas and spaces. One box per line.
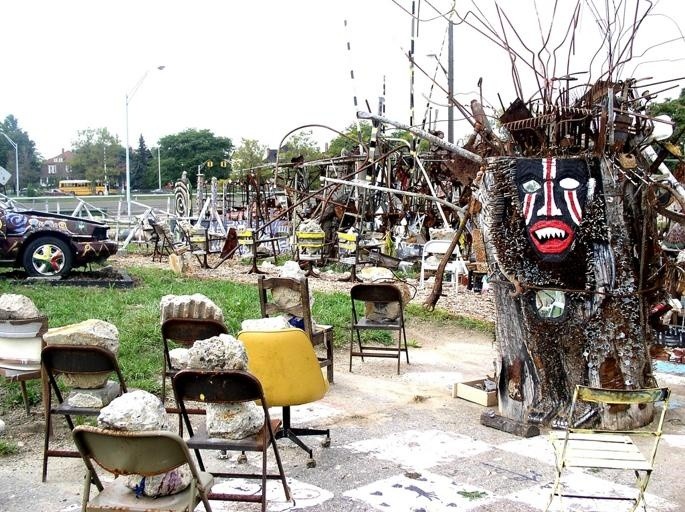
0, 193, 119, 280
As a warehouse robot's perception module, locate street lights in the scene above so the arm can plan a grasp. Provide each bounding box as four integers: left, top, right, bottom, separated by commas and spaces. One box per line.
0, 131, 20, 196
125, 65, 165, 219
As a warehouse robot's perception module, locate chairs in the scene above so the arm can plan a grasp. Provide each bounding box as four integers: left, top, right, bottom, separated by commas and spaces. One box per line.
147, 219, 384, 282
542, 383, 673, 512
236, 330, 334, 468
419, 240, 461, 299
160, 319, 241, 459
172, 370, 295, 509
0, 318, 62, 414
257, 274, 335, 383
40, 345, 129, 485
348, 286, 411, 374
72, 425, 216, 511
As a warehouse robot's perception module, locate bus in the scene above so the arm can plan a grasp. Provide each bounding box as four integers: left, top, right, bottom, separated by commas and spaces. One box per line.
59, 179, 106, 196
